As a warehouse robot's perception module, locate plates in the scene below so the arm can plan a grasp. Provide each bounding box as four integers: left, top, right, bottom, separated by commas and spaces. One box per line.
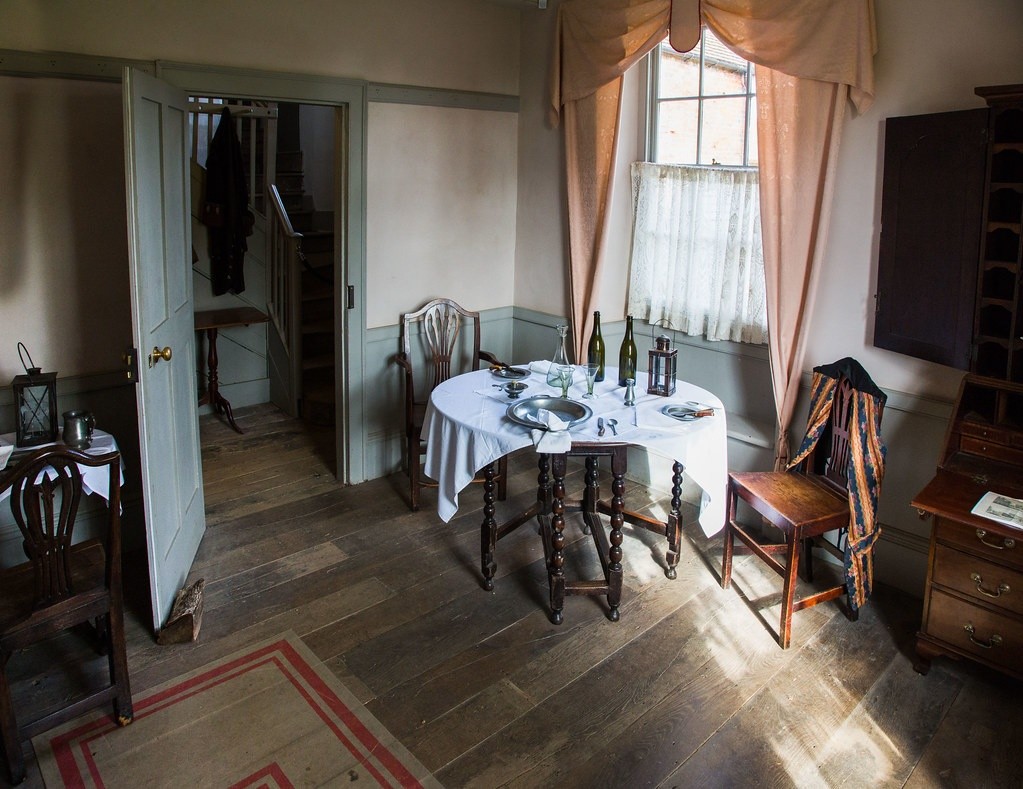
661, 404, 702, 421
490, 368, 531, 381
506, 394, 593, 432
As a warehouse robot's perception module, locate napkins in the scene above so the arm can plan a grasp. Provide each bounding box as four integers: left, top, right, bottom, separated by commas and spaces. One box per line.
530, 428, 572, 454
635, 404, 691, 432
525, 406, 570, 431
530, 359, 560, 373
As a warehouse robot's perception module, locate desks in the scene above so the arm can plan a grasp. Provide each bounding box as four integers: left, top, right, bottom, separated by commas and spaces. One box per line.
193, 306, 269, 435
0, 424, 126, 654
424, 364, 729, 625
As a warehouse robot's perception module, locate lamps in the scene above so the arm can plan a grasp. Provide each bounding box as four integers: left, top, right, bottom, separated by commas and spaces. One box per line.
10, 342, 60, 446
648, 319, 678, 395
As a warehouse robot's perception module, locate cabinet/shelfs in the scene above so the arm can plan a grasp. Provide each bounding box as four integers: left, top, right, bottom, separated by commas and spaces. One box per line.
873, 85, 1023, 676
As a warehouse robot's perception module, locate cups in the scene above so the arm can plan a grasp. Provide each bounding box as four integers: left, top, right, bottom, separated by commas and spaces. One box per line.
62, 410, 96, 450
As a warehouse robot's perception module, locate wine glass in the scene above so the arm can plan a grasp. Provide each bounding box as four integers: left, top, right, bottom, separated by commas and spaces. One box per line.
556, 366, 575, 400
582, 363, 600, 399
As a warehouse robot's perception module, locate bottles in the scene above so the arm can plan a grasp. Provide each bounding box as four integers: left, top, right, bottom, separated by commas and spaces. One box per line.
619, 316, 637, 387
547, 325, 573, 387
588, 311, 605, 382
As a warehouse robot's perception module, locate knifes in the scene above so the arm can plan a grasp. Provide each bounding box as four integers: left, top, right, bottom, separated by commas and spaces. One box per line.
598, 417, 605, 436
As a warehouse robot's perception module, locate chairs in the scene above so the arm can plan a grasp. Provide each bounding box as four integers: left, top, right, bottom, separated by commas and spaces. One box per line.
0, 446, 134, 785
395, 298, 511, 511
721, 357, 888, 650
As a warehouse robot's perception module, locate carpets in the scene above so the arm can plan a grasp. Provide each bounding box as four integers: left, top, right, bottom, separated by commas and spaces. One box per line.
31, 627, 445, 789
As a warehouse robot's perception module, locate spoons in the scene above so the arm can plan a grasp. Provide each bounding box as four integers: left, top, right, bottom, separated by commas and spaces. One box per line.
608, 419, 617, 436
670, 412, 714, 418
